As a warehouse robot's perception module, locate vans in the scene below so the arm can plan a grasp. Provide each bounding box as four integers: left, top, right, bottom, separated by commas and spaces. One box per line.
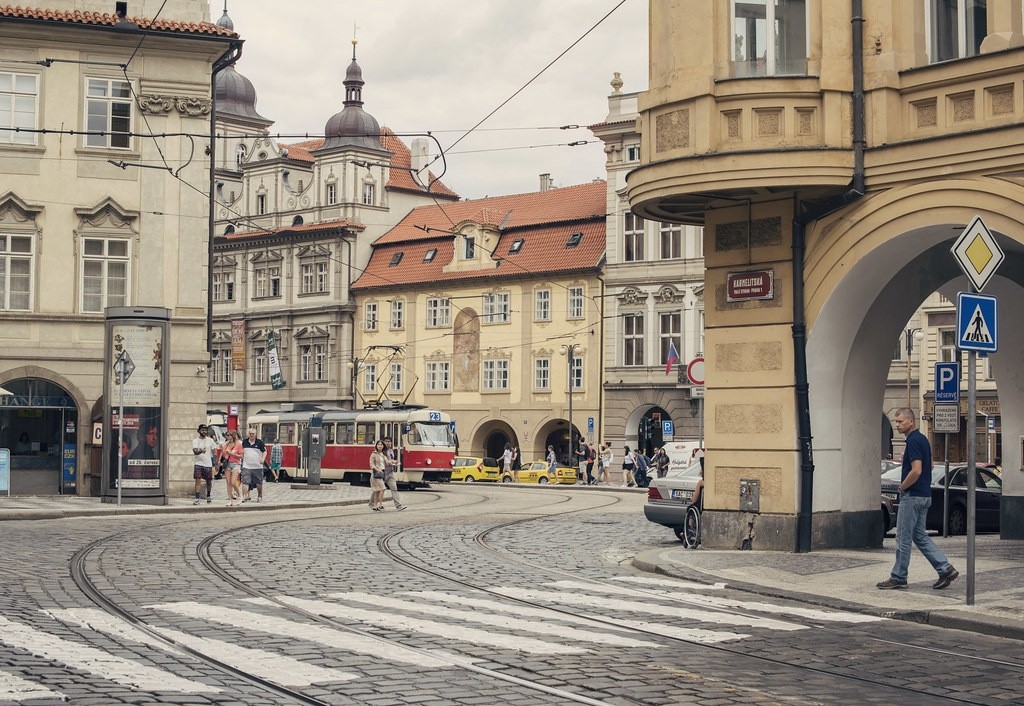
636, 441, 705, 489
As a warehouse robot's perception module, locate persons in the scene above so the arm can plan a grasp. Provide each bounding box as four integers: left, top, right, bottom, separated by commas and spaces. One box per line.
192, 425, 217, 505
368, 436, 407, 512
680, 468, 704, 539
546, 445, 559, 484
122, 421, 159, 479
562, 456, 569, 466
620, 446, 670, 487
498, 442, 521, 483
575, 437, 612, 486
270, 438, 283, 483
877, 409, 959, 589
689, 450, 696, 467
211, 428, 267, 506
993, 457, 1002, 476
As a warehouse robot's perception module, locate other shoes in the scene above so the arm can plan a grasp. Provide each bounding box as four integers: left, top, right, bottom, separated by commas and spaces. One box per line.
226, 500, 233, 505
245, 495, 251, 501
368, 502, 374, 509
633, 484, 638, 488
274, 477, 279, 481
193, 498, 201, 505
591, 479, 598, 484
236, 494, 243, 505
621, 485, 627, 487
397, 505, 407, 511
206, 497, 212, 503
256, 497, 262, 502
373, 508, 381, 511
275, 479, 278, 482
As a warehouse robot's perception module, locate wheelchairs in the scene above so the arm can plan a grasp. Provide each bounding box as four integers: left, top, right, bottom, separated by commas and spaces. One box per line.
682, 486, 705, 551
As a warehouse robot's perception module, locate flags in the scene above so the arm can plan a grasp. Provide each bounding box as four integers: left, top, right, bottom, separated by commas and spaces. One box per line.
666, 344, 679, 375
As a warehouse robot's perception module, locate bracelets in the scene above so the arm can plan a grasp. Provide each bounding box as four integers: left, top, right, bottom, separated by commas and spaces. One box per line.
898, 486, 903, 493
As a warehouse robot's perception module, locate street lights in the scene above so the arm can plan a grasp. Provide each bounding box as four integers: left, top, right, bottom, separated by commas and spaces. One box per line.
558, 343, 582, 466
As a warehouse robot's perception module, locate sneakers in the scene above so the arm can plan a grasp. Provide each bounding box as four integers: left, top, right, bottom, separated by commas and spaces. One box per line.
933, 565, 959, 588
876, 578, 908, 589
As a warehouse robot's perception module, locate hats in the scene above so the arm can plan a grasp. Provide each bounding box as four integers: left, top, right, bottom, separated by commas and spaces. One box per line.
197, 423, 208, 433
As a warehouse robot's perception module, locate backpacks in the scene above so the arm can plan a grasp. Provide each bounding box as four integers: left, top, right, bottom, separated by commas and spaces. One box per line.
589, 447, 596, 460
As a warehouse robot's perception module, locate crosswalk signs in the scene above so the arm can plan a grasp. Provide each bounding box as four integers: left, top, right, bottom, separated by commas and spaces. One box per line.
957, 292, 999, 352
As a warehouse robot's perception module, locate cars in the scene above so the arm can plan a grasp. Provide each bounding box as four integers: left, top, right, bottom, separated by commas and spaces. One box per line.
881, 463, 1003, 538
450, 457, 499, 483
498, 460, 578, 484
644, 457, 897, 541
881, 459, 898, 472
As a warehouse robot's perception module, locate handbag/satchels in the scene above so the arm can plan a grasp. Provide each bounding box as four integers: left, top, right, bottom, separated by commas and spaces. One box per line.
223, 459, 229, 470
374, 468, 386, 479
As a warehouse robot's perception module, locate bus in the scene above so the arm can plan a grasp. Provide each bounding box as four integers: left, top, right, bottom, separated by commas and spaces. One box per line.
245, 399, 458, 491
